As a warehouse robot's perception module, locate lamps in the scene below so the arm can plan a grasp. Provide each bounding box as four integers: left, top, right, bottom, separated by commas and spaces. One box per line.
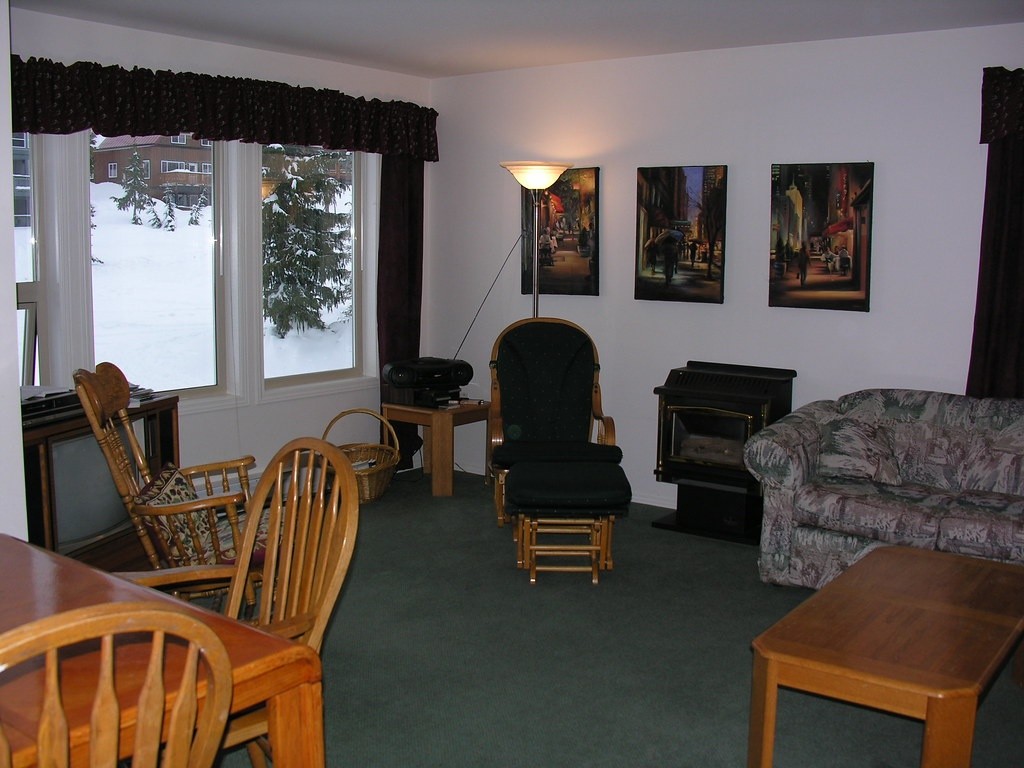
499, 158, 574, 322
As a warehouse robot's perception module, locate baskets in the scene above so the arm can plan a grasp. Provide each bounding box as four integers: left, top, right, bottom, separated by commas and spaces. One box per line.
319, 409, 401, 503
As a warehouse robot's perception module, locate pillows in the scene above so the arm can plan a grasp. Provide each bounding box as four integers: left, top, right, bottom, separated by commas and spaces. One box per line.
817, 415, 901, 483
140, 461, 285, 566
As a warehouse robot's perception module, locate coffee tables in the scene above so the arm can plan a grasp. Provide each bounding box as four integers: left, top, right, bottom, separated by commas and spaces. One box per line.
747, 544, 1024, 767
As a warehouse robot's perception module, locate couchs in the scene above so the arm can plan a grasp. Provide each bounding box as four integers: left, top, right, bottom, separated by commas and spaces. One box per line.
743, 389, 1024, 589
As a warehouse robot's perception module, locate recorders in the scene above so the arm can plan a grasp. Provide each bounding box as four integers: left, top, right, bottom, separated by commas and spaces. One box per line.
382, 357, 474, 389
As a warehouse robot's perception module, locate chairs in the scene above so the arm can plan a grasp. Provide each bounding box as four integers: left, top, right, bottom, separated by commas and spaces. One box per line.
0, 601, 233, 768
72, 362, 296, 630
177, 436, 359, 766
488, 318, 632, 583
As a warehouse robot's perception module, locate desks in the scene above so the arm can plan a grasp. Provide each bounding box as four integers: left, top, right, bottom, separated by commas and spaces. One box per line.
1, 534, 328, 767
21, 384, 179, 556
383, 397, 491, 496
